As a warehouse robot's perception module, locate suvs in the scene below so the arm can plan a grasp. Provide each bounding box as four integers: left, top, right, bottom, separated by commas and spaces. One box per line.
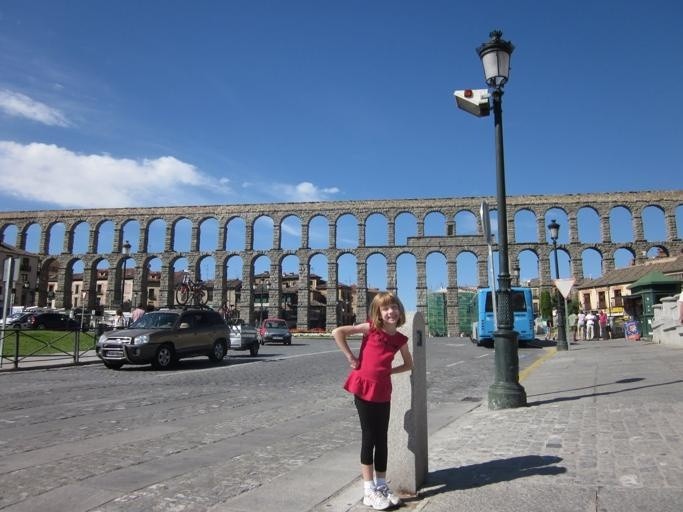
95, 306, 231, 367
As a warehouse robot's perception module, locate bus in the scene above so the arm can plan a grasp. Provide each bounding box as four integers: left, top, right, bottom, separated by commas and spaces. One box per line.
467, 287, 534, 344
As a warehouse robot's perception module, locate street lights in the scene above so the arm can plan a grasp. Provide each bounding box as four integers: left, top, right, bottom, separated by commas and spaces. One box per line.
119, 239, 131, 306
454, 29, 526, 410
546, 217, 567, 350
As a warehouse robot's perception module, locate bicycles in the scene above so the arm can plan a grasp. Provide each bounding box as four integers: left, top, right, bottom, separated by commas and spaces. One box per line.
174, 269, 210, 306
216, 296, 240, 324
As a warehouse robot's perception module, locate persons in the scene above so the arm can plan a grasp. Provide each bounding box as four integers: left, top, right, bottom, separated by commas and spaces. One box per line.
577, 310, 585, 339
130, 302, 144, 322
584, 311, 596, 341
598, 310, 607, 338
567, 310, 579, 342
544, 315, 553, 340
605, 319, 612, 339
113, 310, 125, 331
331, 290, 412, 510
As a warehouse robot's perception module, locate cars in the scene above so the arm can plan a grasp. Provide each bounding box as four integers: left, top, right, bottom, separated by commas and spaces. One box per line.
0, 306, 79, 331
259, 317, 292, 346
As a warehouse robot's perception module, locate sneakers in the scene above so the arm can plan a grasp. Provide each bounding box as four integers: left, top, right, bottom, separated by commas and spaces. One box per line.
376, 482, 401, 506
363, 488, 393, 511
